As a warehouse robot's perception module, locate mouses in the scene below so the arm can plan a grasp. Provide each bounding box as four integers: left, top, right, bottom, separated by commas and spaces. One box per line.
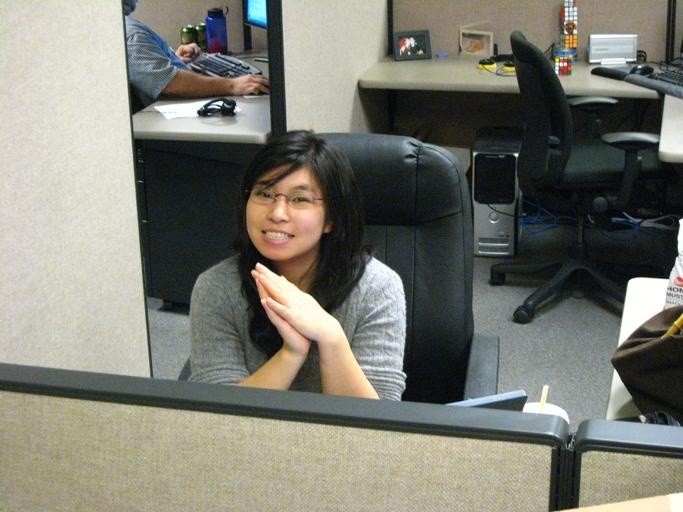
630, 63, 654, 74
248, 90, 269, 96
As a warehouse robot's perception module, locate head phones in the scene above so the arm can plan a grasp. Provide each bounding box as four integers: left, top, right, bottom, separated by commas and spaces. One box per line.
197, 97, 236, 116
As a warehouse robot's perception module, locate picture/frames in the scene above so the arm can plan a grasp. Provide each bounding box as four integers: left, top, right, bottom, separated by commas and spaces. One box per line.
393, 30, 432, 61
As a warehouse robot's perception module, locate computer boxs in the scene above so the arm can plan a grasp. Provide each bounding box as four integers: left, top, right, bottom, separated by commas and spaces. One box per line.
471, 121, 518, 257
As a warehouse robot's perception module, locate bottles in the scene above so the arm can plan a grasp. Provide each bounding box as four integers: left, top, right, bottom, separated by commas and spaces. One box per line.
661, 257, 682, 315
204, 7, 227, 54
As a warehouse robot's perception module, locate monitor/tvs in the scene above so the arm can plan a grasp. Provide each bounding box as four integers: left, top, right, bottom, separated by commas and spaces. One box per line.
242, 0, 272, 30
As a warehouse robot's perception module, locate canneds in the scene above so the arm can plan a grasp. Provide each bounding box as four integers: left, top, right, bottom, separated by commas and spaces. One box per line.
179, 24, 193, 45
194, 22, 208, 52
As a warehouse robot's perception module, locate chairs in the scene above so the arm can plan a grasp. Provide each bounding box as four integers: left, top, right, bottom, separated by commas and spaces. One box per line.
318, 125, 501, 416
488, 32, 683, 324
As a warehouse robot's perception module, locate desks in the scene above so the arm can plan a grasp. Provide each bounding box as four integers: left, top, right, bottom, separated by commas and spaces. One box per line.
657, 89, 683, 169
605, 276, 683, 422
358, 54, 658, 100
130, 91, 271, 315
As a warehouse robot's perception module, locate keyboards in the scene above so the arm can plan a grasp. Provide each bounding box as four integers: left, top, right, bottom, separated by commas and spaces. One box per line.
189, 54, 263, 79
624, 69, 683, 101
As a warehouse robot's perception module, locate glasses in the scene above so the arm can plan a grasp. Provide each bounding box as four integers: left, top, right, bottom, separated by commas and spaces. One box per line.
251, 187, 325, 208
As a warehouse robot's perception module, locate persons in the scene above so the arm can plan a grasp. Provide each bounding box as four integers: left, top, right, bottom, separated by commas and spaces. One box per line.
187, 129, 407, 403
399, 37, 424, 55
122, 0, 271, 107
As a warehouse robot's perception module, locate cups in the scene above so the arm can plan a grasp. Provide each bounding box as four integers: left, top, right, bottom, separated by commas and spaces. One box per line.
524, 400, 568, 421
